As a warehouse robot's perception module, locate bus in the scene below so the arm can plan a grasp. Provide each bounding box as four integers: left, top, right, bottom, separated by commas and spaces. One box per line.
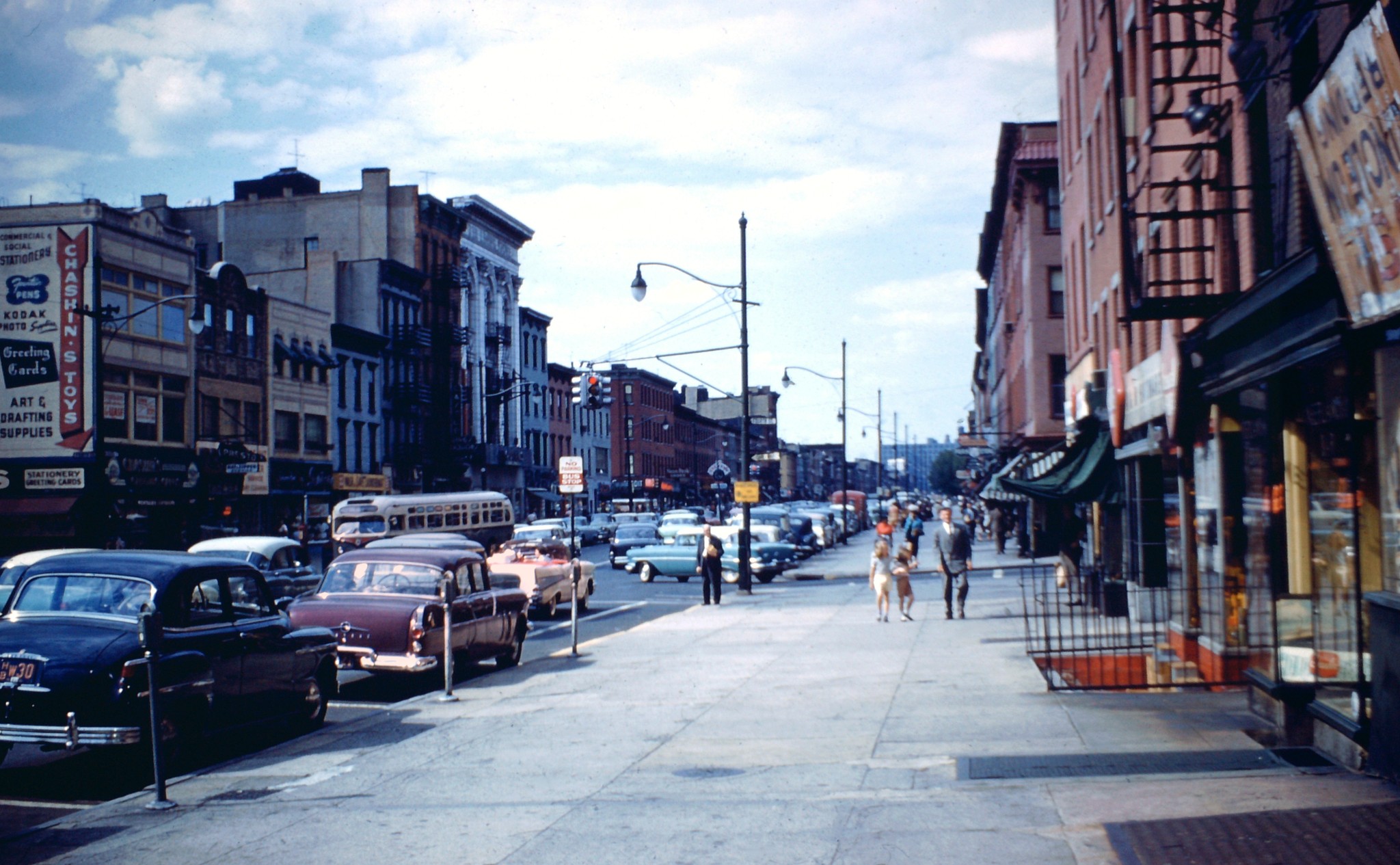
327, 489, 516, 561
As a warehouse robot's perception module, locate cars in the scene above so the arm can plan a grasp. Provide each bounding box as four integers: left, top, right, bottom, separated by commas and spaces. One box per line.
287, 546, 494, 691
0, 546, 342, 801
1162, 488, 1400, 568
0, 548, 219, 615
473, 536, 597, 620
184, 536, 323, 606
511, 523, 583, 558
530, 486, 919, 585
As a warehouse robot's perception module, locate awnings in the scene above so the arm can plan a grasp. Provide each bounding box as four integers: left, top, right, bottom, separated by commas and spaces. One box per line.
980, 451, 1066, 503
531, 490, 567, 502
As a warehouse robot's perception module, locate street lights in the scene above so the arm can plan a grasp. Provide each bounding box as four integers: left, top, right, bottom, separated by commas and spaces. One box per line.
69, 264, 206, 548
624, 400, 670, 512
780, 337, 848, 546
478, 356, 543, 491
631, 211, 752, 593
837, 389, 883, 521
690, 421, 728, 506
861, 411, 898, 500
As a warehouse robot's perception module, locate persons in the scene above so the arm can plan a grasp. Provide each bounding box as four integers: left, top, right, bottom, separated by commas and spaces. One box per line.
696, 523, 724, 605
957, 497, 1008, 554
527, 507, 538, 525
942, 496, 952, 508
934, 506, 972, 620
552, 506, 591, 522
598, 501, 611, 513
891, 548, 919, 621
904, 511, 924, 568
280, 515, 329, 541
1056, 505, 1090, 605
870, 541, 894, 622
876, 495, 934, 549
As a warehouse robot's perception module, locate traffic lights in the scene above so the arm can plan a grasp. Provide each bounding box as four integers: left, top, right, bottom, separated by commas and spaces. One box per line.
600, 373, 611, 405
214, 495, 234, 520
571, 373, 587, 407
585, 374, 603, 406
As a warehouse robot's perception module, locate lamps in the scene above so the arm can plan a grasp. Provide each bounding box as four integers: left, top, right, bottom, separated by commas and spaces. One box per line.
1184, 0, 1351, 135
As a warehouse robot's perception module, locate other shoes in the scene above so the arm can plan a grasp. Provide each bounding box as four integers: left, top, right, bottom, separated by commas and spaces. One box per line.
958, 605, 965, 618
884, 616, 887, 623
904, 610, 913, 621
877, 616, 881, 622
947, 611, 952, 620
901, 614, 907, 621
